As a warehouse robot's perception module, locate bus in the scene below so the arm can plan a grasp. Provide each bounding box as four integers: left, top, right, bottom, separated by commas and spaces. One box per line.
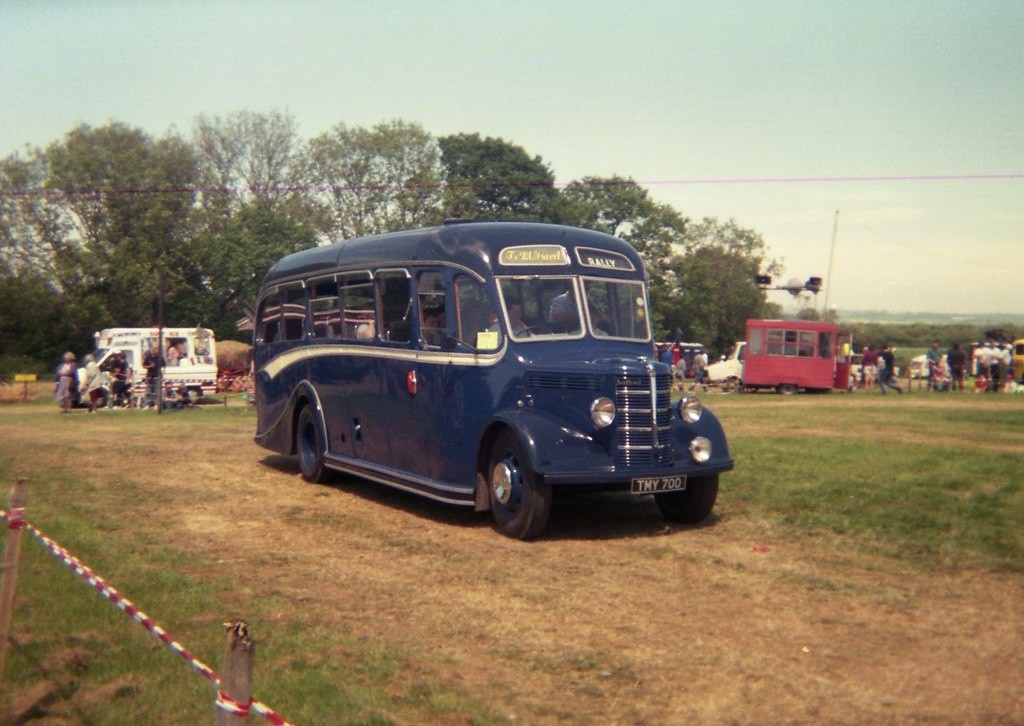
253, 223, 734, 540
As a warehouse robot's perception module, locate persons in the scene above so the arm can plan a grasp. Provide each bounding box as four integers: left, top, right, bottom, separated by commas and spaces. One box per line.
549, 282, 604, 325
84, 354, 102, 412
973, 341, 1011, 392
423, 304, 446, 344
54, 352, 79, 412
862, 344, 903, 395
168, 342, 185, 366
927, 342, 967, 391
143, 348, 166, 410
357, 305, 375, 340
488, 296, 532, 342
102, 353, 141, 410
663, 346, 709, 392
162, 382, 193, 408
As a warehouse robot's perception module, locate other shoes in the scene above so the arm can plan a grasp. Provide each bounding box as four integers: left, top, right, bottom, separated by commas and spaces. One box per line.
883, 392, 887, 394
899, 387, 902, 394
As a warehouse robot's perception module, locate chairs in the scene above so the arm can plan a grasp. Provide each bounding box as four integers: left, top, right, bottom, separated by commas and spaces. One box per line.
385, 319, 413, 344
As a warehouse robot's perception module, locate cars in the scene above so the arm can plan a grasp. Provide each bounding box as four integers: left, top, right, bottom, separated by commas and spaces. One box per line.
1012, 340, 1024, 384
707, 341, 746, 384
849, 354, 877, 382
910, 355, 950, 379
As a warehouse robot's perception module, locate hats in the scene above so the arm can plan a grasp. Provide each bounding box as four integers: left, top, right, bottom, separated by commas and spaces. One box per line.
699, 349, 705, 354
973, 342, 1013, 350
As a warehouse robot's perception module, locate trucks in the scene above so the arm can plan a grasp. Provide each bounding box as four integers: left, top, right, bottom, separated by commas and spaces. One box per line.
73, 327, 218, 409
